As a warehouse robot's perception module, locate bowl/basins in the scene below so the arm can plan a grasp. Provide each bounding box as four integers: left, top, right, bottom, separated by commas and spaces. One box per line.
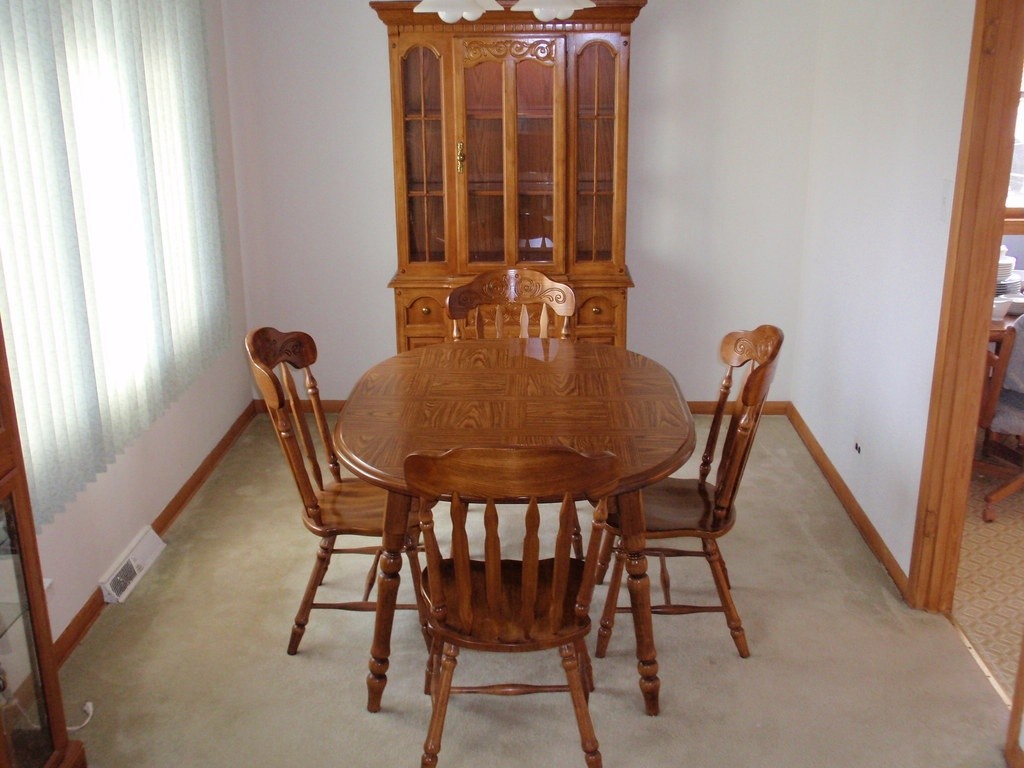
990, 297, 1012, 322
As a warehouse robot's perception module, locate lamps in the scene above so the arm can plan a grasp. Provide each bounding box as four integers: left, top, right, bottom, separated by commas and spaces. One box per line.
414, 0, 597, 22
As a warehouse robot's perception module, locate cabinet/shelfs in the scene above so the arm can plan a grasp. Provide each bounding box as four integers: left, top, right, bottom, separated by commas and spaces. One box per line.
368, 0, 644, 355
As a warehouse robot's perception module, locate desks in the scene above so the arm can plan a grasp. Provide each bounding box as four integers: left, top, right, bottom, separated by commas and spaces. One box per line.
978, 314, 1019, 356
324, 332, 702, 721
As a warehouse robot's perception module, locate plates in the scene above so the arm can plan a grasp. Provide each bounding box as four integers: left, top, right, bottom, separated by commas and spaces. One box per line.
995, 255, 1024, 316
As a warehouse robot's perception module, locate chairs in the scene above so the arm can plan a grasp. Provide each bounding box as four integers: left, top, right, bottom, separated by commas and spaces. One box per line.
584, 320, 784, 660
400, 436, 621, 768
445, 261, 577, 342
973, 429, 1024, 523
241, 325, 454, 657
